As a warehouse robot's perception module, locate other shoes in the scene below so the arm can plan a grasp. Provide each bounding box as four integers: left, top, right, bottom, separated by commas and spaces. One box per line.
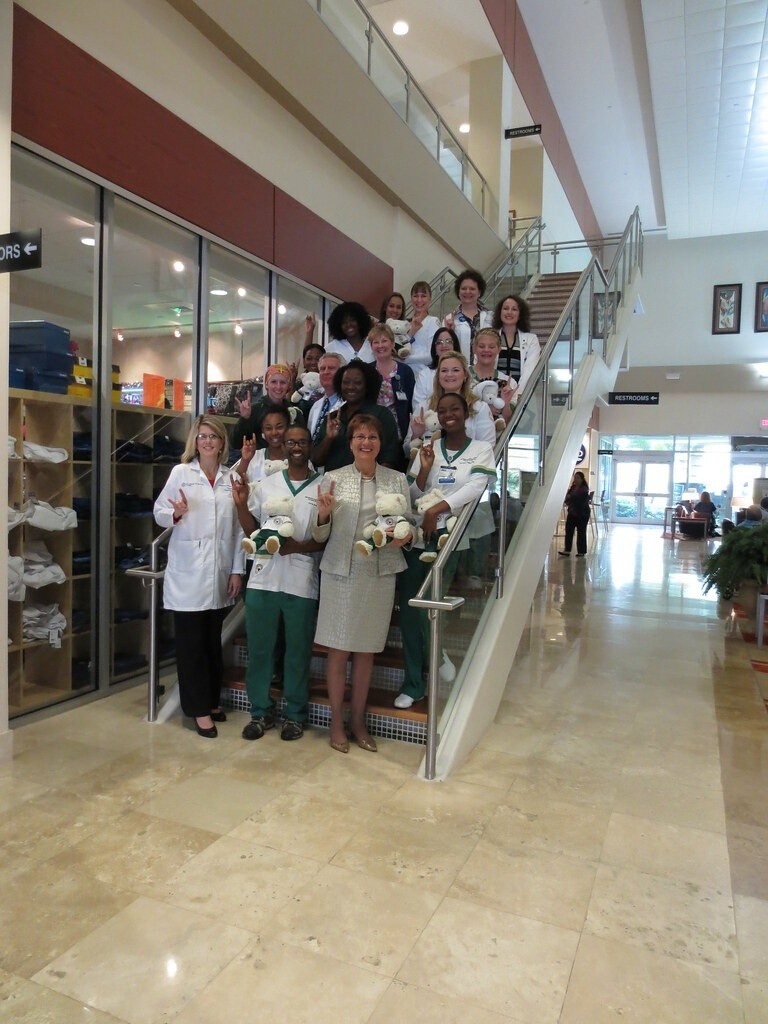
559, 552, 570, 556
466, 576, 484, 591
394, 692, 424, 709
576, 554, 585, 556
439, 649, 457, 683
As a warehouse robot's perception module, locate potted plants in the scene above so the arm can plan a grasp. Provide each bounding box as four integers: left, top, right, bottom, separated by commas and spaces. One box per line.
699, 519, 768, 644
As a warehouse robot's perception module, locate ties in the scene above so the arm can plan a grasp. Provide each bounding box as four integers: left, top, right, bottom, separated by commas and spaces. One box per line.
313, 399, 329, 446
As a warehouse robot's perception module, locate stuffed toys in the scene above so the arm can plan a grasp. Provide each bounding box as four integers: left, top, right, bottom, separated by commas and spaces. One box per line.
355, 490, 411, 558
290, 371, 325, 403
247, 458, 288, 493
385, 317, 413, 359
416, 488, 458, 562
409, 409, 441, 459
242, 495, 295, 555
472, 380, 506, 431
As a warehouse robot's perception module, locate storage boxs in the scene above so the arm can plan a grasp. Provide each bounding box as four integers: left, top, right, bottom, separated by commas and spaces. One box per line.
9, 320, 69, 354
9, 352, 75, 394
67, 355, 122, 403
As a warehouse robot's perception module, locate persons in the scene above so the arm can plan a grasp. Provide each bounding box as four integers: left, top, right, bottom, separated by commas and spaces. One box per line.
694, 491, 717, 537
153, 415, 246, 738
737, 505, 762, 529
499, 488, 524, 522
230, 425, 322, 741
558, 472, 590, 556
222, 269, 541, 710
489, 493, 500, 521
760, 497, 768, 519
310, 413, 412, 753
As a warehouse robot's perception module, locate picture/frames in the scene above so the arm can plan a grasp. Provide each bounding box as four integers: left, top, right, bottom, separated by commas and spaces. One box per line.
592, 290, 620, 339
712, 283, 742, 334
754, 282, 768, 332
558, 296, 579, 341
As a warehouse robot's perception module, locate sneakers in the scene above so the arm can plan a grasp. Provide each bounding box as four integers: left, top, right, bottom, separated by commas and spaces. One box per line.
243, 714, 304, 741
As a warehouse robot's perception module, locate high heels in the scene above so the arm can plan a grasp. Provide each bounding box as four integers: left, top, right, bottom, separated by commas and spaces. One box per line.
330, 721, 377, 753
194, 710, 226, 739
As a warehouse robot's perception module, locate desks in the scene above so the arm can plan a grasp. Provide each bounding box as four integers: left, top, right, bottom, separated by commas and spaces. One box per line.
671, 515, 708, 539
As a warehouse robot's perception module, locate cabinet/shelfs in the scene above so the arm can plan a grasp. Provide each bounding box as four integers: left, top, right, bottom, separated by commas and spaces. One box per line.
7, 388, 243, 718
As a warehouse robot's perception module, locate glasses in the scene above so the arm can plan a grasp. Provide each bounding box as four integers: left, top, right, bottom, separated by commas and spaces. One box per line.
434, 340, 453, 345
197, 434, 221, 440
283, 440, 310, 448
